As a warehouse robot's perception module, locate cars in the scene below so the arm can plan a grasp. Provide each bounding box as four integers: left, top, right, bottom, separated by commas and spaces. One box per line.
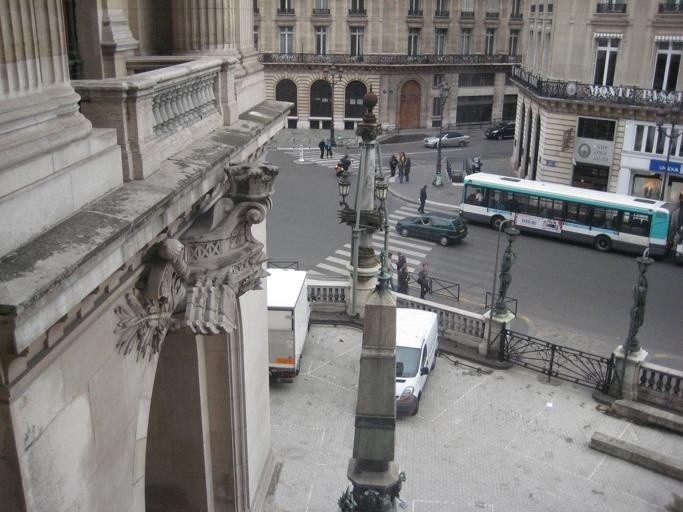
675, 235, 683, 262
424, 132, 470, 148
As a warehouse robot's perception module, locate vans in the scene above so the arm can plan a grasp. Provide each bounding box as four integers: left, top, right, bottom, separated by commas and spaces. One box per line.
394, 308, 439, 416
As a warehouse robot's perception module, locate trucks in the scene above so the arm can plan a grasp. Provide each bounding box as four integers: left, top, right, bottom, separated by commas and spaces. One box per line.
266, 267, 317, 385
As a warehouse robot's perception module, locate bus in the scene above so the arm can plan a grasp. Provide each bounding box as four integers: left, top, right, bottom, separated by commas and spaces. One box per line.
459, 172, 680, 256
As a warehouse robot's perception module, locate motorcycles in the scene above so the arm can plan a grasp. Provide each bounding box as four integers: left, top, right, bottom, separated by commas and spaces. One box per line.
472, 153, 482, 173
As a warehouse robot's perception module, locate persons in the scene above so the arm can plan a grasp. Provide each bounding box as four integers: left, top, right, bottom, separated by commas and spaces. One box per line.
386, 152, 411, 184
540, 204, 548, 217
643, 184, 652, 199
416, 183, 427, 215
384, 251, 409, 295
325, 139, 332, 159
418, 261, 430, 299
316, 137, 324, 159
474, 189, 483, 203
472, 158, 482, 173
420, 218, 431, 226
511, 200, 519, 208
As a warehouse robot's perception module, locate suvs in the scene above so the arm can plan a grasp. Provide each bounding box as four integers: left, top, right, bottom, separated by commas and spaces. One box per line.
484, 121, 515, 140
395, 211, 468, 247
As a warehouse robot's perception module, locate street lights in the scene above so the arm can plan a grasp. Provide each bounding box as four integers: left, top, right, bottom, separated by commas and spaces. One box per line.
338, 84, 388, 318
432, 79, 451, 187
657, 99, 683, 201
318, 62, 345, 149
623, 243, 654, 352
492, 216, 520, 315
